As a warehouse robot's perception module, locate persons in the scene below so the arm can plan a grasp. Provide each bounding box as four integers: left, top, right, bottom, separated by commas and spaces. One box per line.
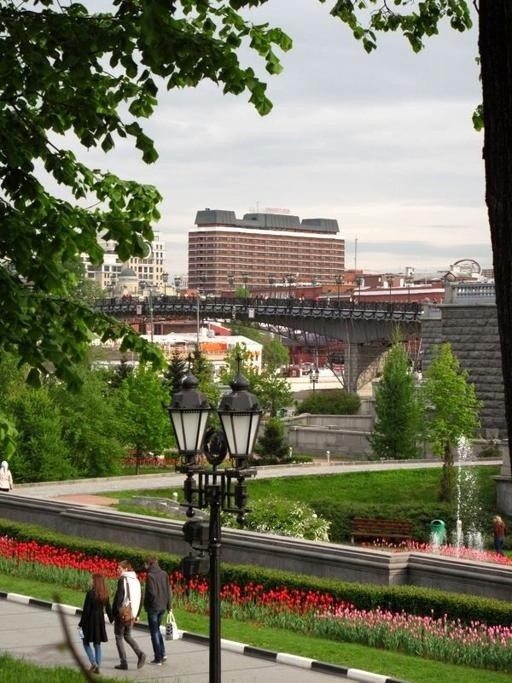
77, 574, 113, 673
111, 560, 145, 669
144, 556, 173, 666
494, 516, 506, 556
0, 461, 13, 492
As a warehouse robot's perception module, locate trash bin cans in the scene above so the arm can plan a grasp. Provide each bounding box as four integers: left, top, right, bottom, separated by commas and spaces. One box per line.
430, 520, 446, 545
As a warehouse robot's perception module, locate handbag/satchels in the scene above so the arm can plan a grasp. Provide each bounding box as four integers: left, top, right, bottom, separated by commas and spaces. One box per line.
118, 603, 135, 621
164, 613, 181, 641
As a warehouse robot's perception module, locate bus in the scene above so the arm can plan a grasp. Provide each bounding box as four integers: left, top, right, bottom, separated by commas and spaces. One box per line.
332, 349, 345, 376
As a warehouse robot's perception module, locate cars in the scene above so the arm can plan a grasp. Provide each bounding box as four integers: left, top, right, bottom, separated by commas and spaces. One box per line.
279, 360, 316, 378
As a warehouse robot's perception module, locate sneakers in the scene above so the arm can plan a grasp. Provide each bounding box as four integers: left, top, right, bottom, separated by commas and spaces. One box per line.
135, 652, 146, 669
114, 663, 129, 670
150, 653, 168, 666
87, 661, 100, 674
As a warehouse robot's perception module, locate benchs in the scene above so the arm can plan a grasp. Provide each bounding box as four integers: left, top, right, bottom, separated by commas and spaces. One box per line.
347, 515, 418, 547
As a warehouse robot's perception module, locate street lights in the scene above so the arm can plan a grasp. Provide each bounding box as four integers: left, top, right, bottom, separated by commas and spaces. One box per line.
308, 367, 319, 392
148, 287, 161, 345
194, 292, 207, 354
158, 344, 270, 683
